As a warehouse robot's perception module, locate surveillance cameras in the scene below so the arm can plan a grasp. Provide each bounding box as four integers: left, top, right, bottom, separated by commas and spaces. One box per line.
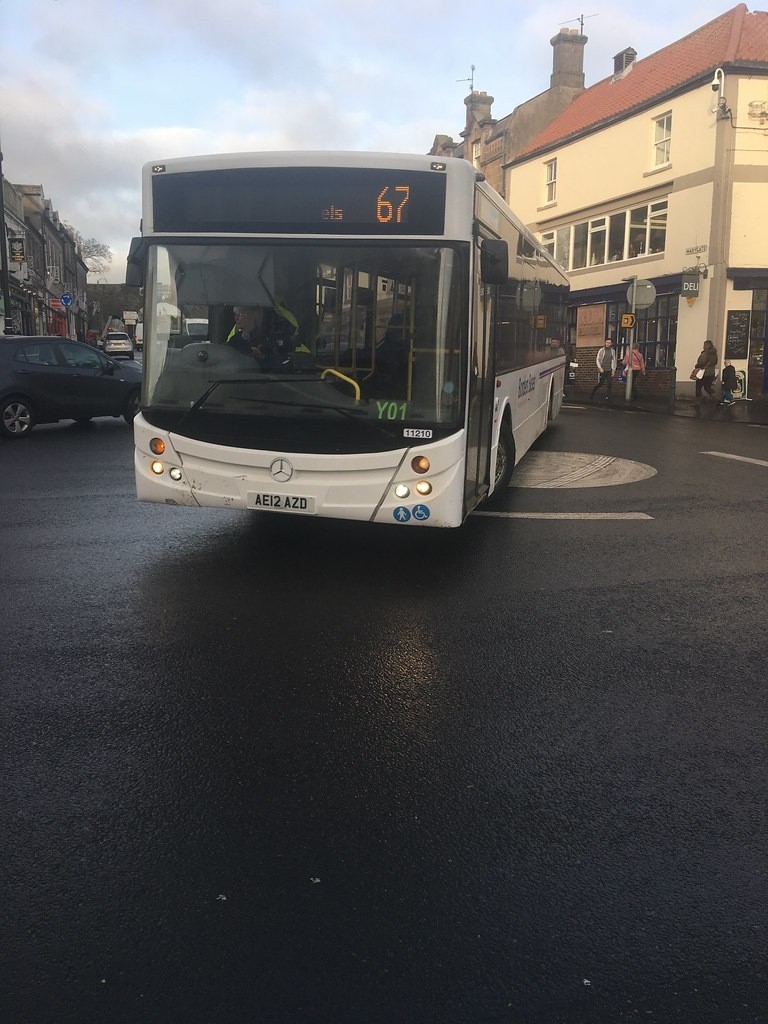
711, 79, 720, 92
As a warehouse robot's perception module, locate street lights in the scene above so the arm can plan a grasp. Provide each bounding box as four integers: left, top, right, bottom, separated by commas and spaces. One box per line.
622, 274, 638, 402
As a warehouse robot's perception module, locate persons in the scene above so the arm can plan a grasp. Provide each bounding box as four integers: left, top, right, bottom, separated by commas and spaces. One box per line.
77, 329, 93, 346
220, 305, 294, 375
65, 343, 84, 366
374, 314, 409, 376
547, 338, 565, 358
621, 343, 645, 400
721, 360, 737, 406
590, 338, 618, 401
690, 340, 720, 407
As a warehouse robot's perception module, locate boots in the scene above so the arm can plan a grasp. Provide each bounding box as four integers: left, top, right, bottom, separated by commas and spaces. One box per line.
711, 393, 720, 407
690, 396, 702, 407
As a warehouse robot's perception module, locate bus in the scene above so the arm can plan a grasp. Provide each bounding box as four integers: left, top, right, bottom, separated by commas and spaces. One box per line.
123, 146, 575, 528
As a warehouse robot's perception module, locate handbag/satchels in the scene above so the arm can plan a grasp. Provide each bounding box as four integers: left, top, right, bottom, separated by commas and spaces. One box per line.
618, 371, 627, 383
711, 369, 719, 385
690, 368, 705, 380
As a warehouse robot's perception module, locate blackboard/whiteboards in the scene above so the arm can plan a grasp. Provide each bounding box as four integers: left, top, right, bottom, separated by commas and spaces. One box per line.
724, 310, 751, 360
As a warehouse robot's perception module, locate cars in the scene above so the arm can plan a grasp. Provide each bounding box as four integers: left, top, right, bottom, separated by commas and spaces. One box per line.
0, 333, 145, 439
101, 331, 135, 360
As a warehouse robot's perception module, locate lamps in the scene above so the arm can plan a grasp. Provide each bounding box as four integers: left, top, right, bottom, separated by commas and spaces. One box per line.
711, 68, 731, 119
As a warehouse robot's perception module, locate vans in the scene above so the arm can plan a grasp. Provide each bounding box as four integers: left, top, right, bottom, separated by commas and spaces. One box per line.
133, 322, 143, 352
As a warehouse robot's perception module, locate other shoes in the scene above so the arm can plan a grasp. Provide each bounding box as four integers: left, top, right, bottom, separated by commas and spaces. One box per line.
606, 397, 611, 401
728, 400, 736, 407
720, 401, 724, 405
590, 395, 594, 401
